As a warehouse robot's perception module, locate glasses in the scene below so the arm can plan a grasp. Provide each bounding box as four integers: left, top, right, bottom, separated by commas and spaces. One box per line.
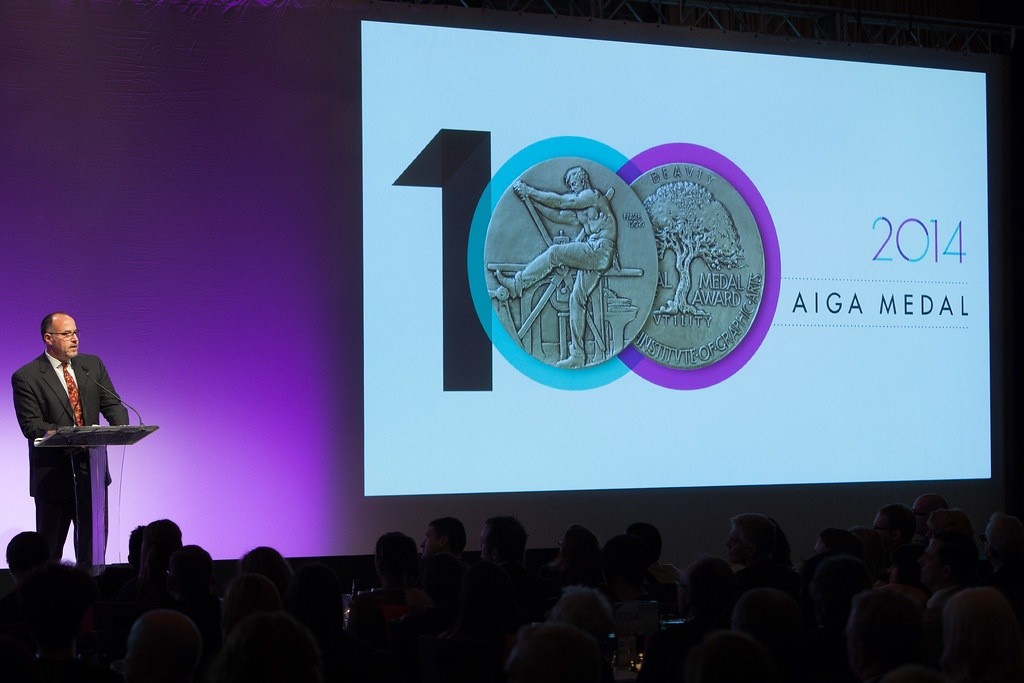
49, 329, 81, 339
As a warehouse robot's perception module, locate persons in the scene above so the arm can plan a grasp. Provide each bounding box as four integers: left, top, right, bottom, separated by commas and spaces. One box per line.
11, 312, 129, 565
0, 493, 1024, 683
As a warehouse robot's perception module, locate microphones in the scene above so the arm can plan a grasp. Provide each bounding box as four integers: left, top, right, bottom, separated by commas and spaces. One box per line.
72, 365, 143, 427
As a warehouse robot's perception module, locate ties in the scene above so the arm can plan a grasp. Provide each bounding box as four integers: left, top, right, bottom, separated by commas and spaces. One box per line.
61, 360, 84, 427
